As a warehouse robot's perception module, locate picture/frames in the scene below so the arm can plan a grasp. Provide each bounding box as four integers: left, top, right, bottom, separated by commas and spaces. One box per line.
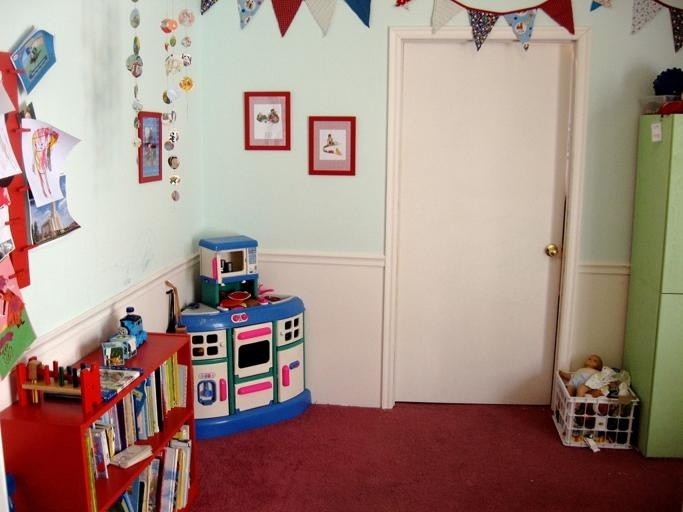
309, 116, 356, 176
245, 92, 290, 150
138, 111, 162, 184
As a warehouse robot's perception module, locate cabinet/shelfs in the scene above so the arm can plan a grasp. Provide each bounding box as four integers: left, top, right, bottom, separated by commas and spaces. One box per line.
622, 114, 683, 458
1, 333, 197, 512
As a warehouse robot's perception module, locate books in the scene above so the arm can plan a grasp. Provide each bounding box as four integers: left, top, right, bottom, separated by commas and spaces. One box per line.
85, 352, 193, 512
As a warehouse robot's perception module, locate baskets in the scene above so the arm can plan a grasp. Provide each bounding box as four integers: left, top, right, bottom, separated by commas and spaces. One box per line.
552, 372, 640, 449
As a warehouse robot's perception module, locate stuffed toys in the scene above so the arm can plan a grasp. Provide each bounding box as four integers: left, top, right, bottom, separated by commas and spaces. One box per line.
557, 355, 631, 454
